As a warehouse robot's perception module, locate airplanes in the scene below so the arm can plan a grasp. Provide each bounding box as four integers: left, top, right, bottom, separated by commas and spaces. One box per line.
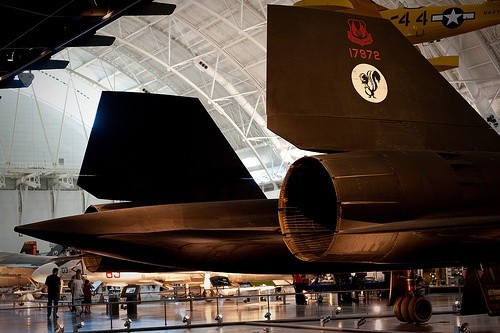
0, 254, 315, 302
13, 1, 499, 332
292, 0, 498, 75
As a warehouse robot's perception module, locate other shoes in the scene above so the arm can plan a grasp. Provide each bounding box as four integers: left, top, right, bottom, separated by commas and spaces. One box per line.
53, 314, 59, 318
47, 313, 51, 317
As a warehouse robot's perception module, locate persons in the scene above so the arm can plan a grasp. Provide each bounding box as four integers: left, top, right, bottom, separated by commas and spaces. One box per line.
45, 268, 61, 318
68, 269, 94, 316
291, 274, 320, 305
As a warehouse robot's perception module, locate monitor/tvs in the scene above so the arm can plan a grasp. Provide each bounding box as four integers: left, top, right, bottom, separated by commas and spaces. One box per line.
125, 287, 137, 293
